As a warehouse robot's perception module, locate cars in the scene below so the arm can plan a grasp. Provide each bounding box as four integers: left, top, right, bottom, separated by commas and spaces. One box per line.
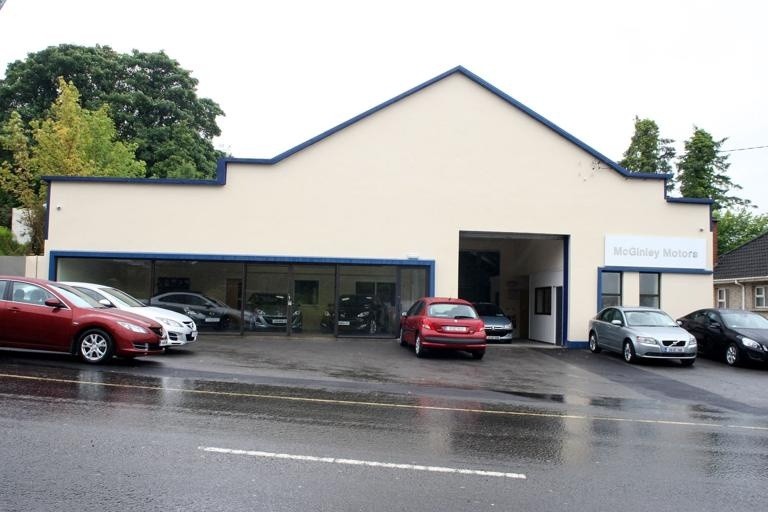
471, 302, 513, 344
2, 278, 169, 364
20, 281, 199, 346
246, 291, 303, 333
320, 294, 389, 336
398, 296, 487, 360
585, 303, 767, 373
136, 291, 256, 332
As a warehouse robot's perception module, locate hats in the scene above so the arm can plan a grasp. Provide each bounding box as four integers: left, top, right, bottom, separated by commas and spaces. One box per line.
585, 303, 767, 373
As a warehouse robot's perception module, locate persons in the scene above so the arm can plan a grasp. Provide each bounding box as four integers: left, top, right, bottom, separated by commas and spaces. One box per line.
29, 290, 42, 304
